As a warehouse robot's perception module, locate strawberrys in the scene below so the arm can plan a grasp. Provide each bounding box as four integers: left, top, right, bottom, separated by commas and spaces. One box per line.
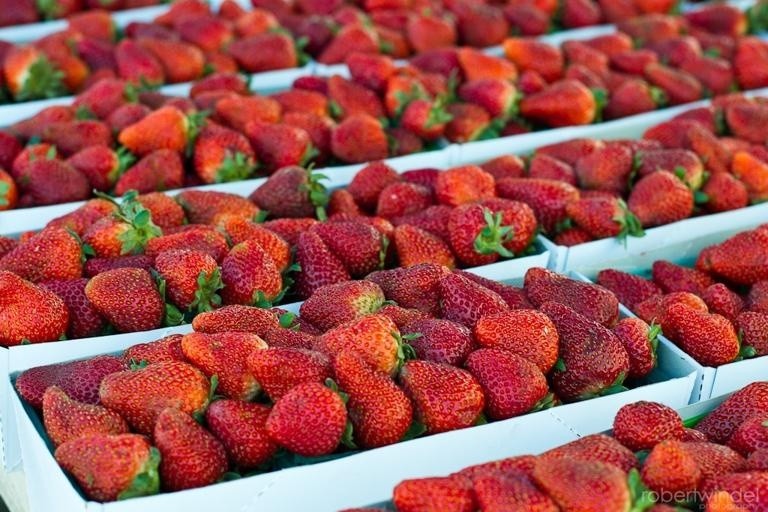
0, 0, 768, 511
